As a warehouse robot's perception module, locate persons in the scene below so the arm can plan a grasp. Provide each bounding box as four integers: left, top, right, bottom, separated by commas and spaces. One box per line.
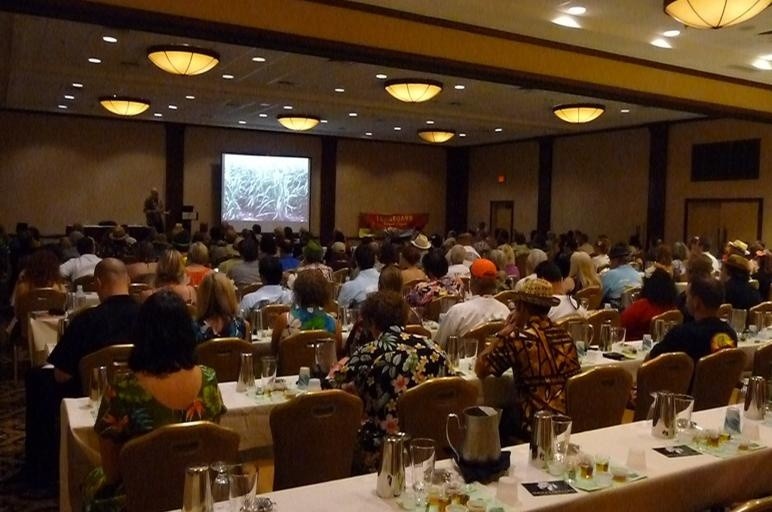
474, 277, 582, 445
644, 272, 738, 397
2, 187, 772, 499
80, 288, 226, 511
321, 291, 453, 474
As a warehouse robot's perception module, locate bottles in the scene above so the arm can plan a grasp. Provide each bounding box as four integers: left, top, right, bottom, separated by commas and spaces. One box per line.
600, 323, 612, 353
88, 366, 110, 406
743, 377, 766, 419
723, 408, 741, 434
446, 336, 459, 367
376, 433, 405, 498
575, 340, 586, 359
182, 464, 212, 511
530, 410, 555, 470
648, 390, 677, 438
298, 366, 311, 390
237, 352, 256, 393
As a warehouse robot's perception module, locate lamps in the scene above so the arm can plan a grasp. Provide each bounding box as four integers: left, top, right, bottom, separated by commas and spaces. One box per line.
417, 128, 458, 145
382, 77, 447, 105
147, 45, 221, 78
275, 113, 323, 133
96, 95, 152, 118
551, 102, 607, 127
660, 1, 772, 30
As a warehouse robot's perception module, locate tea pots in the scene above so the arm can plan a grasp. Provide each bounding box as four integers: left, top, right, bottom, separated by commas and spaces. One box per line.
307, 338, 339, 374
446, 406, 504, 464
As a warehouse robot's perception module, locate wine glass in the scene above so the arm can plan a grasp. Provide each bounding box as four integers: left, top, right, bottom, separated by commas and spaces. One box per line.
211, 461, 230, 512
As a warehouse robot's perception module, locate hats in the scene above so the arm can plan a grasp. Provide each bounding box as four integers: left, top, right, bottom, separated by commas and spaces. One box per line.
110, 225, 128, 241
507, 277, 560, 307
303, 240, 323, 257
411, 233, 432, 250
331, 242, 345, 254
720, 254, 752, 271
728, 239, 751, 254
470, 259, 496, 278
608, 243, 631, 258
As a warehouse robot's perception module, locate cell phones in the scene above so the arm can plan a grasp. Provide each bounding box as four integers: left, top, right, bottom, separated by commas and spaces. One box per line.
603, 352, 624, 360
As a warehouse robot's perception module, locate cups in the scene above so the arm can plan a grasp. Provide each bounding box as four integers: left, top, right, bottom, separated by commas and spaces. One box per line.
462, 338, 478, 369
675, 394, 695, 431
258, 358, 277, 400
228, 463, 259, 512
547, 415, 574, 477
611, 327, 626, 354
410, 437, 437, 492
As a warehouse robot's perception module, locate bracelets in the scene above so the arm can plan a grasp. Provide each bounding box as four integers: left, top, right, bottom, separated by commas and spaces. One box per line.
495, 333, 505, 340
